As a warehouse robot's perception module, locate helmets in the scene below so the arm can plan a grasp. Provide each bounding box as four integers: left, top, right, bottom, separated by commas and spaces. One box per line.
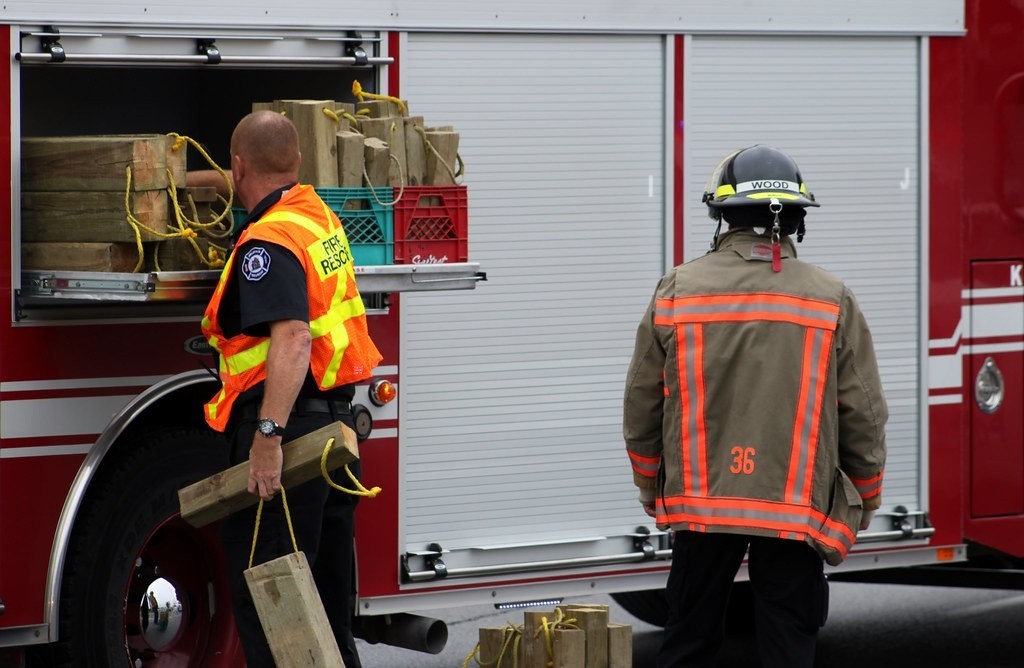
704, 145, 820, 225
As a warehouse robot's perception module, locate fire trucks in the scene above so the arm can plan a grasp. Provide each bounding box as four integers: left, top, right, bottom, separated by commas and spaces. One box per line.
0, 0, 1024, 668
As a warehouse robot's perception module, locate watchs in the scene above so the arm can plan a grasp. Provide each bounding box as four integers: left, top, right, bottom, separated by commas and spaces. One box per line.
257, 419, 285, 437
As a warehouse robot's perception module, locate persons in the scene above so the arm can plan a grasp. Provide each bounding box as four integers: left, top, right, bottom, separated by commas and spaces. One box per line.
624, 144, 889, 630
186, 111, 382, 668
147, 591, 178, 633
